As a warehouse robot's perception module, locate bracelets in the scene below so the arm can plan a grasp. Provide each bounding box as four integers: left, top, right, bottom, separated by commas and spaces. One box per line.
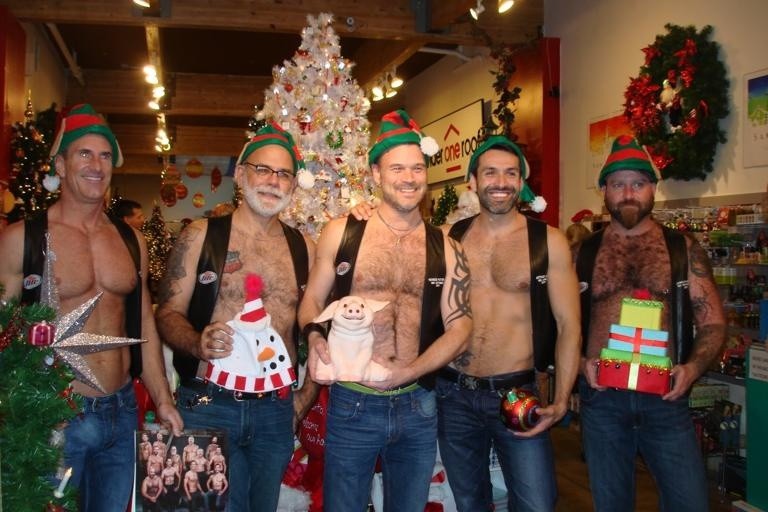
301, 323, 328, 341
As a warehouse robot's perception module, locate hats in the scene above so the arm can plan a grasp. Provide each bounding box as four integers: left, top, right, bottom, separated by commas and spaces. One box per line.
369, 110, 439, 169
599, 135, 661, 185
236, 116, 315, 191
465, 136, 548, 215
41, 104, 124, 192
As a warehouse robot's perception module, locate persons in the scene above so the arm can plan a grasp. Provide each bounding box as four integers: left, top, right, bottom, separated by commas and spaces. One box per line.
294, 110, 473, 511
116, 198, 145, 231
0, 103, 185, 511
432, 136, 585, 511
571, 134, 731, 511
143, 118, 321, 511
136, 428, 230, 511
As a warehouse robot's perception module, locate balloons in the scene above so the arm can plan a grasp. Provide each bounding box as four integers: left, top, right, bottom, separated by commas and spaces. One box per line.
157, 155, 207, 209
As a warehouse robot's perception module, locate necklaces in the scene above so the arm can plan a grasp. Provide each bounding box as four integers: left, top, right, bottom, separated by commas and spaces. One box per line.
378, 206, 424, 245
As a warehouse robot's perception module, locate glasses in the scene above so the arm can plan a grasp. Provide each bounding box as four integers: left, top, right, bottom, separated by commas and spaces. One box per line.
245, 162, 296, 184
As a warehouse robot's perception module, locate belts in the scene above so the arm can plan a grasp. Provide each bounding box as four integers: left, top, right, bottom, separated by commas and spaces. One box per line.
190, 378, 297, 404
439, 366, 536, 393
